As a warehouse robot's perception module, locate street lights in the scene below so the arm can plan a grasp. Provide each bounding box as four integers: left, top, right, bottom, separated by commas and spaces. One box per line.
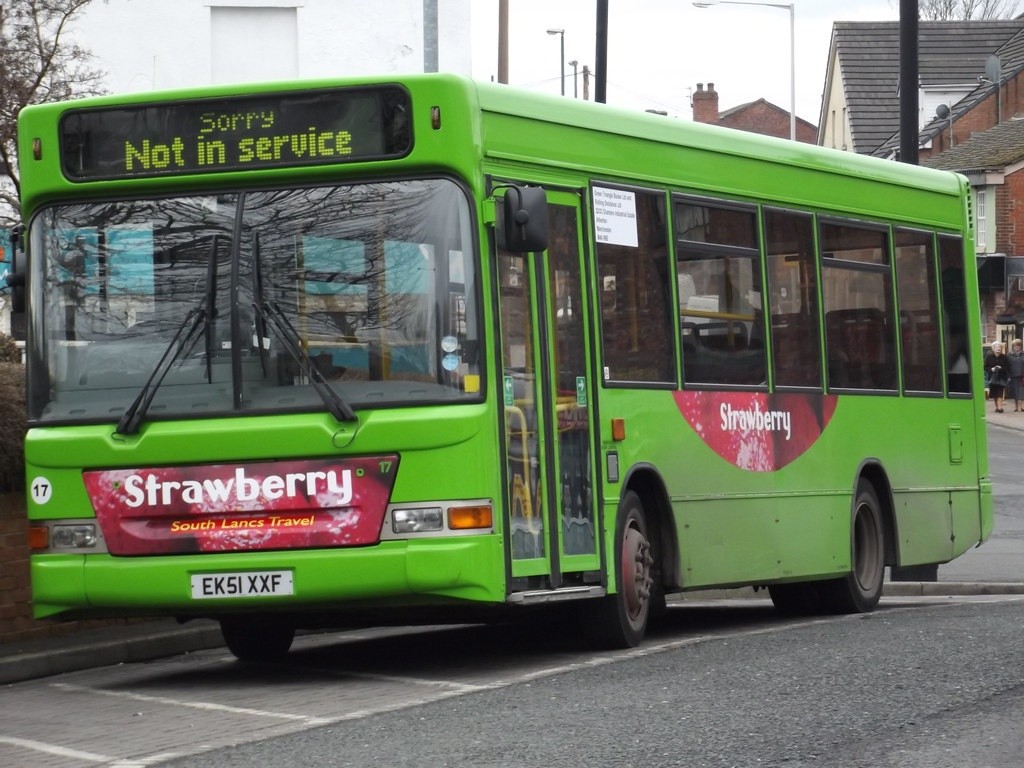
568, 60, 579, 99
547, 27, 567, 96
694, 0, 795, 141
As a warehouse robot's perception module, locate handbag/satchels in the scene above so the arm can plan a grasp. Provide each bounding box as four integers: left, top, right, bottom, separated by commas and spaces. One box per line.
988, 368, 1008, 388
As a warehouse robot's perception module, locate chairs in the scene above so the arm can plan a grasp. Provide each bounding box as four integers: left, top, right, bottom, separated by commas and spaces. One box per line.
497, 300, 953, 389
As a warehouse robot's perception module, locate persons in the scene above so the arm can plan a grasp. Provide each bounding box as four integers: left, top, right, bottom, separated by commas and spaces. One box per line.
1007, 338, 1024, 412
984, 340, 1010, 413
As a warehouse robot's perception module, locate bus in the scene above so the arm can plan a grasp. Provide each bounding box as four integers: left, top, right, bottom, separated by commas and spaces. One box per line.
5, 71, 996, 665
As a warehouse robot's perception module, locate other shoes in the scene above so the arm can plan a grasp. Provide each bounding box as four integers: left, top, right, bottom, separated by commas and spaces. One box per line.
995, 408, 1004, 413
1014, 410, 1018, 412
1021, 408, 1024, 412
